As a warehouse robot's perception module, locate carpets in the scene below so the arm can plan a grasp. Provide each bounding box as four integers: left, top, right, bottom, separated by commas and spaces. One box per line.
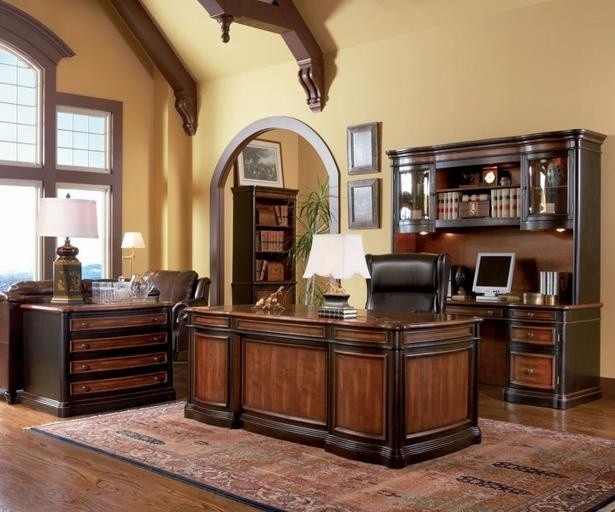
24, 396, 615, 512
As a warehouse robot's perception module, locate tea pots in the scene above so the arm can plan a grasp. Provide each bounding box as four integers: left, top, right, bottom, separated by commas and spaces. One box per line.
116, 274, 155, 299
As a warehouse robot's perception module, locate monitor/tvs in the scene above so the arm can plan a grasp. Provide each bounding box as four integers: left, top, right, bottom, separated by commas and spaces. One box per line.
471, 252, 516, 300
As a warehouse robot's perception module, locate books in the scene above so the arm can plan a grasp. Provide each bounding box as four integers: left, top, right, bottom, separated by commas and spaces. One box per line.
435, 186, 524, 221
254, 195, 293, 282
316, 304, 358, 320
538, 269, 572, 295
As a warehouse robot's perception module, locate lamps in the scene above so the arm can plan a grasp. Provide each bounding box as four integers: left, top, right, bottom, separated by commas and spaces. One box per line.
302, 233, 371, 287
121, 231, 145, 280
38, 193, 98, 304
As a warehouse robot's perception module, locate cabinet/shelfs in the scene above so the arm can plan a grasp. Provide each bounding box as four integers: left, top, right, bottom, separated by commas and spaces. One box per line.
446, 301, 603, 410
385, 128, 607, 303
230, 185, 299, 304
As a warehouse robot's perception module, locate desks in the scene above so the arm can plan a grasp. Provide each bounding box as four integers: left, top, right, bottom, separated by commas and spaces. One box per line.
183, 304, 483, 469
20, 302, 177, 418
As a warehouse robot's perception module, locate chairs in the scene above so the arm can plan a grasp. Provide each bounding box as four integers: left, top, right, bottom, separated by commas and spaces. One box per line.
363, 253, 451, 313
141, 269, 211, 361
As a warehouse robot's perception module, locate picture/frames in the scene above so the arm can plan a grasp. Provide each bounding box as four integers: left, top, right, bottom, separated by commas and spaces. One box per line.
346, 122, 380, 175
346, 178, 379, 229
234, 138, 284, 189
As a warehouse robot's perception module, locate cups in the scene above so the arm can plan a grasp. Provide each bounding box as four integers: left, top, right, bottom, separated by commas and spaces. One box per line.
91, 282, 119, 305
